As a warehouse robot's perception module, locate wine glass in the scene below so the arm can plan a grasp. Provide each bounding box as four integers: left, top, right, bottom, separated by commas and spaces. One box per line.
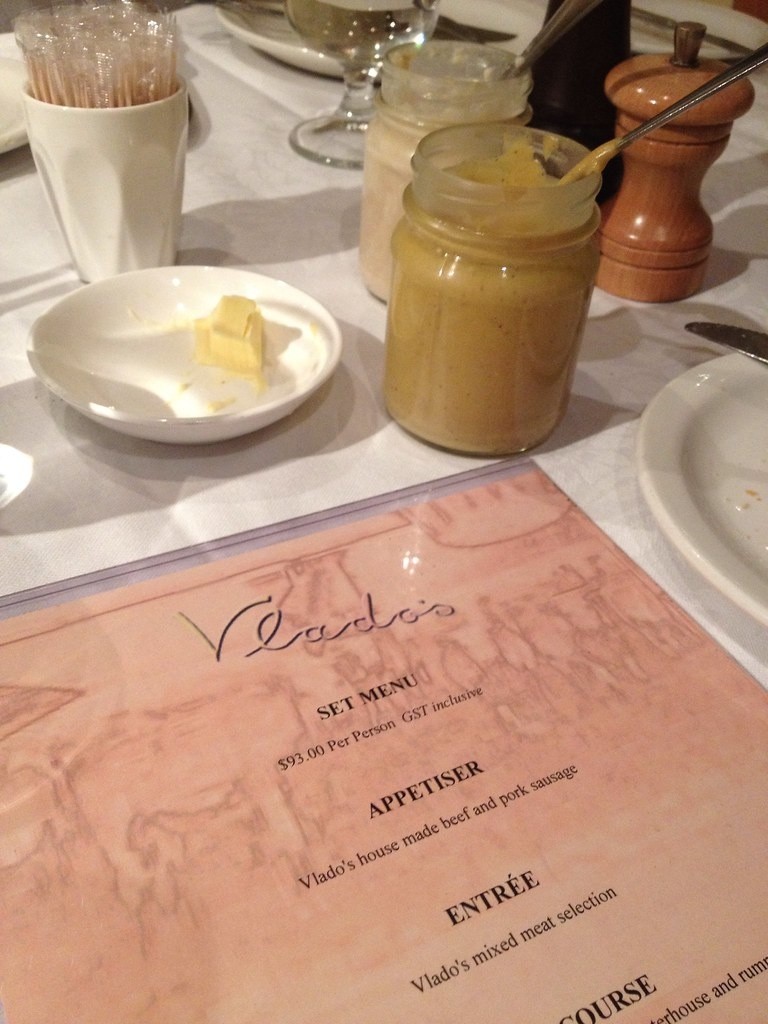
286, 1, 442, 170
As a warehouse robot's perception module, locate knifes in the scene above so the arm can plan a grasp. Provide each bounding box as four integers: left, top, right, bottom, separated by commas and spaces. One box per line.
684, 321, 768, 366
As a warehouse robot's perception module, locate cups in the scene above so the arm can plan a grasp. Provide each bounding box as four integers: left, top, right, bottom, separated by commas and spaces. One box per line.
360, 44, 535, 304
381, 122, 603, 460
19, 74, 190, 282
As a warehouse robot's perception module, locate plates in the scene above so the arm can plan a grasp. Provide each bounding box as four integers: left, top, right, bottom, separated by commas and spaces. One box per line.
26, 266, 342, 444
635, 350, 768, 626
213, 0, 343, 78
